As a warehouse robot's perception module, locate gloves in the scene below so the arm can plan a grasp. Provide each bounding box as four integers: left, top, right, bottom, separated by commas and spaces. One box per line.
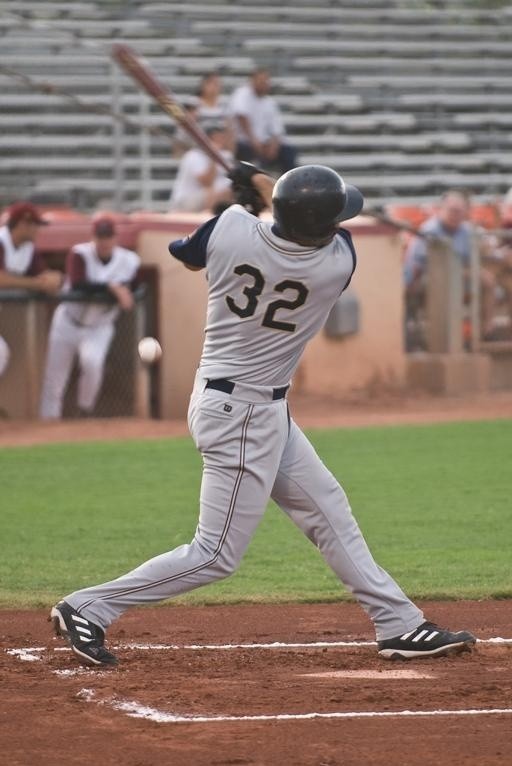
225, 158, 267, 214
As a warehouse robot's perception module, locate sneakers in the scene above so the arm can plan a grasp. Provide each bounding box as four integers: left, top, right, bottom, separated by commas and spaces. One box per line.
374, 616, 476, 662
46, 599, 118, 668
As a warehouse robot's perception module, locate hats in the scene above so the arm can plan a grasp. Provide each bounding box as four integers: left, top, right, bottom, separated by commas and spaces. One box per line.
90, 215, 116, 240
0, 200, 50, 228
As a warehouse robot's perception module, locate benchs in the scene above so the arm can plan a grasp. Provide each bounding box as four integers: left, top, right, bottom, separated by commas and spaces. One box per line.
0, 2, 511, 210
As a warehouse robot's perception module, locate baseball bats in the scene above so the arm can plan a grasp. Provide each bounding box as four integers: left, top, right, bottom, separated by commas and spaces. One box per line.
112, 43, 231, 172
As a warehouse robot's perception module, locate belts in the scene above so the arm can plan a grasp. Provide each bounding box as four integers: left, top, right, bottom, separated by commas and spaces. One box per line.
201, 377, 290, 402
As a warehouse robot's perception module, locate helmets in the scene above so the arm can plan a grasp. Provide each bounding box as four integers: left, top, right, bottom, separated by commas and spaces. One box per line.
269, 163, 366, 246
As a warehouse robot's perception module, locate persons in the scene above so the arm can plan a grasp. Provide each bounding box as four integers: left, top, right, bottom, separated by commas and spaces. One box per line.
494, 188, 512, 297
403, 188, 497, 341
49, 159, 478, 669
1, 203, 63, 420
170, 65, 295, 212
41, 213, 146, 423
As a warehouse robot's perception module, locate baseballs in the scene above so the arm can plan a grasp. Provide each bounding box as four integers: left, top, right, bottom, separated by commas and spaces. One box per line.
137, 334, 164, 366
